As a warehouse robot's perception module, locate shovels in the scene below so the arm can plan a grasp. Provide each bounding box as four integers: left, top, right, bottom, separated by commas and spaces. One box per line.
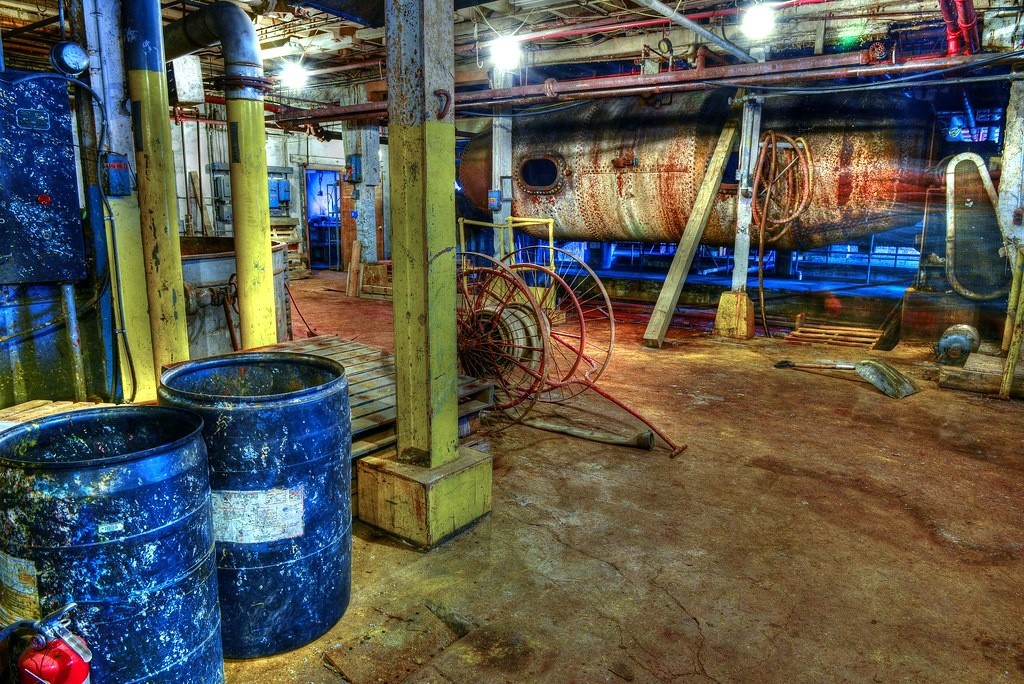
775, 359, 921, 401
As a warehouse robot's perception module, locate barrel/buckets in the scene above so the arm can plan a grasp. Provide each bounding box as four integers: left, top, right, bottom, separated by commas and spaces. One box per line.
0, 404, 228, 684
159, 353, 353, 660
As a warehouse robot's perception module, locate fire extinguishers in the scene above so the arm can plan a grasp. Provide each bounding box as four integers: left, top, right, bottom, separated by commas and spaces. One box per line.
0, 602, 91, 684
